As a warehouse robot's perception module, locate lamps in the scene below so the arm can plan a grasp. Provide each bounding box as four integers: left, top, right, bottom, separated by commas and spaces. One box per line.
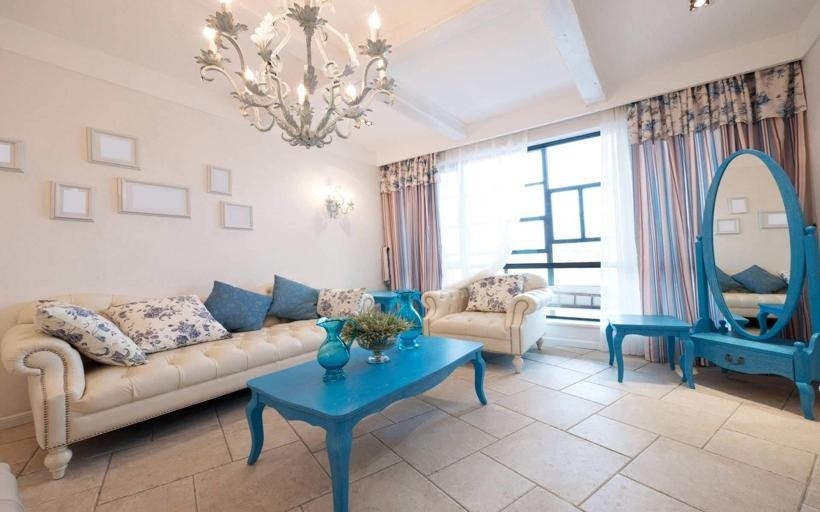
325, 195, 354, 219
193, 0, 396, 149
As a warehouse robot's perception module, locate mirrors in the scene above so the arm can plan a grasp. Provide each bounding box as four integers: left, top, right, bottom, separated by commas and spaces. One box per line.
702, 149, 806, 340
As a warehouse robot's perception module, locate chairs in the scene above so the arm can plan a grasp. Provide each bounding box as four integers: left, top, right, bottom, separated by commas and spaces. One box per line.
421, 273, 553, 374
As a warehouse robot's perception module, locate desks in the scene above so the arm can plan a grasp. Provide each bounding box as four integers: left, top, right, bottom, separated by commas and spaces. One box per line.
373, 291, 399, 312
246, 335, 487, 512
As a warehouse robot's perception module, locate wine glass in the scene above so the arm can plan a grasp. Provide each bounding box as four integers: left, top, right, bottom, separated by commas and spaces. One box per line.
357, 337, 395, 364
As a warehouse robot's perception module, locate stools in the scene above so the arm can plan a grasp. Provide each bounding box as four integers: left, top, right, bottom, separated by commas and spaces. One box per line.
606, 313, 696, 388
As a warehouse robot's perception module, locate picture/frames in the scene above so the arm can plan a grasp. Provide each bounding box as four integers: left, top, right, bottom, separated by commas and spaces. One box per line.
760, 210, 788, 229
220, 200, 253, 229
87, 127, 139, 169
0, 136, 25, 172
50, 181, 95, 221
714, 218, 739, 234
728, 198, 748, 214
207, 165, 232, 195
117, 176, 191, 217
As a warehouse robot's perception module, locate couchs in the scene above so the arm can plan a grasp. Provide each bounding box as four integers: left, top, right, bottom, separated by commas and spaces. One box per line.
722, 291, 786, 319
1, 288, 374, 480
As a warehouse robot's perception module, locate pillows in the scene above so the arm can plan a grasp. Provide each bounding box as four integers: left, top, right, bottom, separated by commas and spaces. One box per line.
33, 300, 148, 367
732, 264, 787, 293
204, 281, 272, 332
268, 274, 319, 319
715, 265, 741, 293
316, 287, 366, 320
99, 295, 232, 354
465, 275, 526, 313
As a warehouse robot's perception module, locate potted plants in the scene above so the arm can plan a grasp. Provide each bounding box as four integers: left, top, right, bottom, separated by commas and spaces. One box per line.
344, 310, 418, 364
